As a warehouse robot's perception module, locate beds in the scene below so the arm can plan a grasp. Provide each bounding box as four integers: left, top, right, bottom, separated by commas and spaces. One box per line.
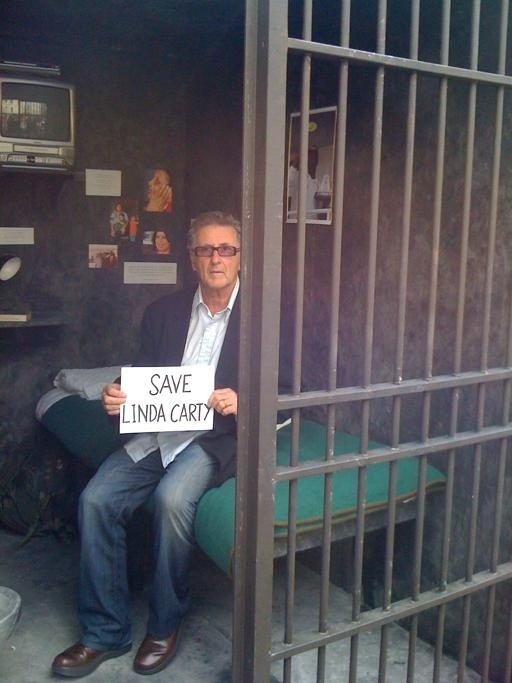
34, 388, 449, 588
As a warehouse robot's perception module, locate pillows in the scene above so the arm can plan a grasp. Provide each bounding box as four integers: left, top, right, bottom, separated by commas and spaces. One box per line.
53, 364, 134, 401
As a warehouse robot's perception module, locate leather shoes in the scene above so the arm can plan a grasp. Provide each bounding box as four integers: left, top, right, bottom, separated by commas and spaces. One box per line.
52, 637, 132, 677
134, 608, 185, 675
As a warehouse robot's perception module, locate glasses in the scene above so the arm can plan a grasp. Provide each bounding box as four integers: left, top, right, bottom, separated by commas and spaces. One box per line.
189, 244, 240, 258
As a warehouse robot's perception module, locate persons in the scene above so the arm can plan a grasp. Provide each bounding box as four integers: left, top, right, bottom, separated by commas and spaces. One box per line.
52, 210, 291, 677
102, 170, 172, 268
287, 146, 320, 221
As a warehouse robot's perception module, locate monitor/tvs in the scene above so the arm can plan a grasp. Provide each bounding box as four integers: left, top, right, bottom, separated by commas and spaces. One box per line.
0, 71, 76, 172
